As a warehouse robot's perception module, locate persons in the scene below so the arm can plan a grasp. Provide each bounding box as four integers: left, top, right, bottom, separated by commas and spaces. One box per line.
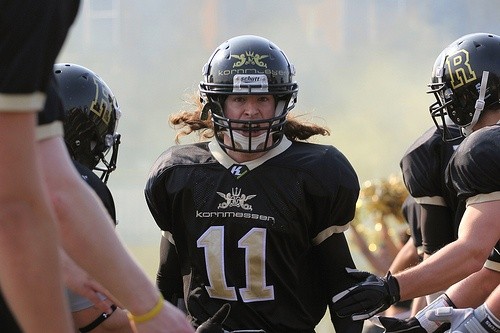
0, 0, 500, 333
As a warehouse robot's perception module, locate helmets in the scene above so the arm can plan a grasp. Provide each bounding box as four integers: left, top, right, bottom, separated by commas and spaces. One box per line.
430, 33, 500, 127
197, 34, 298, 143
53, 63, 122, 169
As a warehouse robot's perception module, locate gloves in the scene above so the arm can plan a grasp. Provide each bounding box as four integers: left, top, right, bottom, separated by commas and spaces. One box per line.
369, 293, 457, 333
425, 306, 500, 333
331, 267, 401, 321
196, 302, 266, 333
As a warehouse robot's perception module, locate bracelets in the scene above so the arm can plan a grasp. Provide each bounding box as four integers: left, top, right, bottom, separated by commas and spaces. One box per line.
132, 293, 165, 322
